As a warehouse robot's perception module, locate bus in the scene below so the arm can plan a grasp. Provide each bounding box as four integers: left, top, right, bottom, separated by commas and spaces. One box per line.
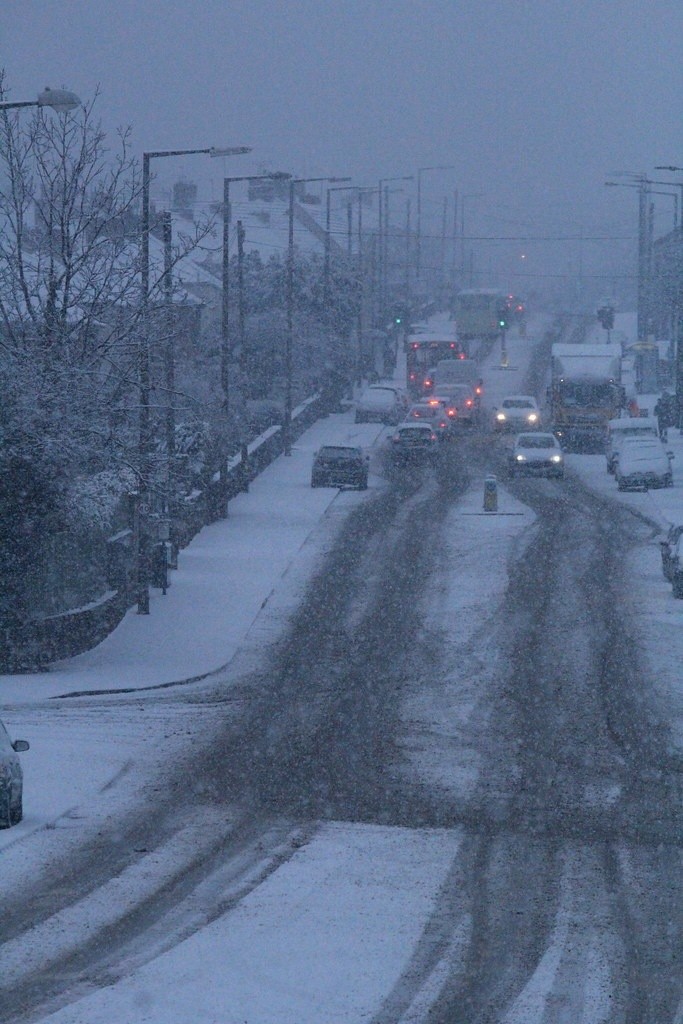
405, 337, 465, 399
456, 289, 501, 339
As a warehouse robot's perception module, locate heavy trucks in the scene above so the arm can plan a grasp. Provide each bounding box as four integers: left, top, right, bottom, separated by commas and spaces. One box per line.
544, 340, 627, 451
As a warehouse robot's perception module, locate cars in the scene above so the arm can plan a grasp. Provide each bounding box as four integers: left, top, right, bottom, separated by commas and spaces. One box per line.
611, 436, 676, 490
404, 397, 456, 441
433, 383, 476, 422
492, 395, 542, 432
507, 432, 566, 479
0, 719, 30, 829
661, 524, 683, 598
356, 385, 407, 425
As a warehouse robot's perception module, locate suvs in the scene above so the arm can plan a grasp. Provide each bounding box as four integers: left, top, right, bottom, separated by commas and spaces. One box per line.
387, 422, 439, 459
311, 444, 369, 490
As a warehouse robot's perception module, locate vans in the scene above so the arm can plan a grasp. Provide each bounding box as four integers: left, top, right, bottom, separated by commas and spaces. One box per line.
604, 419, 664, 473
427, 359, 482, 389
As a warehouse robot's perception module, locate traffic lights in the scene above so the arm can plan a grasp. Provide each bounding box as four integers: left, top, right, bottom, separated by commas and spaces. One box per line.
499, 313, 505, 329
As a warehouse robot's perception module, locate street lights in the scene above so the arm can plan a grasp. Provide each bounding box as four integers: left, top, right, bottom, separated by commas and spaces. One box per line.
324, 166, 484, 408
222, 171, 295, 516
285, 177, 353, 457
138, 145, 255, 614
